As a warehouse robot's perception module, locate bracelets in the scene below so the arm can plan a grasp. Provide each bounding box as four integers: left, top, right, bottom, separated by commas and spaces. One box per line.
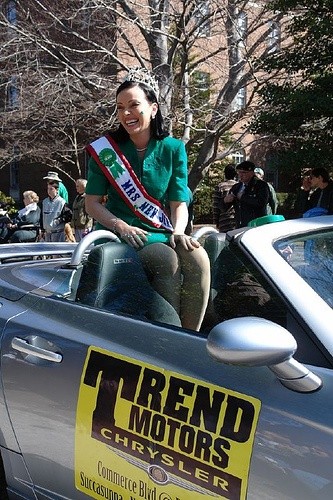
112, 218, 120, 234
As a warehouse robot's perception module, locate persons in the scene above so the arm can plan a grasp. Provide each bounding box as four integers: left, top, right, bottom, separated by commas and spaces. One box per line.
294, 166, 333, 218
217, 161, 277, 232
84, 65, 212, 331
0, 172, 94, 243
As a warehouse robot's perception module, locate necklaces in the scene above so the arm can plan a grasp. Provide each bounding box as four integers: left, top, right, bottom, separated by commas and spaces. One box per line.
136, 148, 145, 152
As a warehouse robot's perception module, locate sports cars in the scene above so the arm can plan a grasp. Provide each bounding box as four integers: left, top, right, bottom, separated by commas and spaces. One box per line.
0, 215, 333, 500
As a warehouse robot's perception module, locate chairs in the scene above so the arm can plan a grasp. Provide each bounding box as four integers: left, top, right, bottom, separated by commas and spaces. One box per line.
76, 243, 183, 328
205, 231, 228, 306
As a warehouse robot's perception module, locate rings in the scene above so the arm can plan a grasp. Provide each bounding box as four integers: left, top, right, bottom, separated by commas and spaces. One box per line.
128, 235, 132, 238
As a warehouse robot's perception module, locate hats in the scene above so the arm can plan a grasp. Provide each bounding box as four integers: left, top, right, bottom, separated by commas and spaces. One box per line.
236, 161, 255, 172
43, 172, 62, 182
